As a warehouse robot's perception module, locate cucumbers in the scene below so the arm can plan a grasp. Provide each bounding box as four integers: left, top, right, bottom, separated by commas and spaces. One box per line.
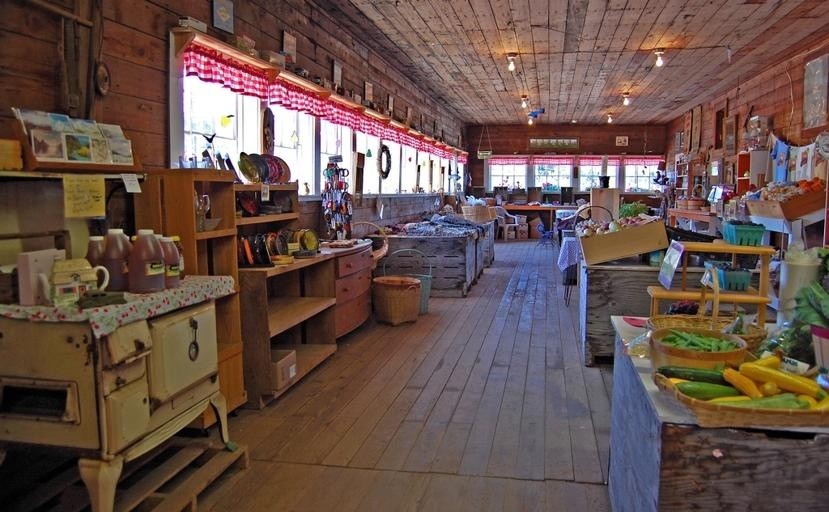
657, 356, 829, 410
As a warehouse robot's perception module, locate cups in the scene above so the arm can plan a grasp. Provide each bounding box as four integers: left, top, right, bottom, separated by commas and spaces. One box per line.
36, 255, 110, 308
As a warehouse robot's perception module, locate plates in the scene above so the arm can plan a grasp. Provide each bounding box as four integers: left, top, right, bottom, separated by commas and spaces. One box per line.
238, 227, 320, 268
237, 151, 292, 182
236, 193, 293, 215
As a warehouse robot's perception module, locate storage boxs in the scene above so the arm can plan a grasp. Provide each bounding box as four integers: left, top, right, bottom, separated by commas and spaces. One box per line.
517, 214, 545, 240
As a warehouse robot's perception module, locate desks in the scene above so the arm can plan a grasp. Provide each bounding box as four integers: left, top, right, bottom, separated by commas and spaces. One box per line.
668, 207, 716, 237
0, 276, 241, 511
498, 204, 578, 232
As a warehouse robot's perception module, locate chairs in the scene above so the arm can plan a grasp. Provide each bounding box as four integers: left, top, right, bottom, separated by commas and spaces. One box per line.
493, 206, 520, 241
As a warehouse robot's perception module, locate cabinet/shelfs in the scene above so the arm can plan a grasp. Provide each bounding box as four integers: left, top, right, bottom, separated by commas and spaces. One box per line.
736, 151, 773, 197
135, 167, 338, 409
674, 163, 695, 201
648, 239, 774, 337
320, 239, 374, 338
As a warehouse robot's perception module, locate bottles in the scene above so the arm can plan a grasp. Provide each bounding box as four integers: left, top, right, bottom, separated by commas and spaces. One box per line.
84, 228, 185, 295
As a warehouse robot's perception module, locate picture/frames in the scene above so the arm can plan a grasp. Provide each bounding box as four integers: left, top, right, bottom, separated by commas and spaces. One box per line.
799, 47, 829, 138
723, 115, 737, 157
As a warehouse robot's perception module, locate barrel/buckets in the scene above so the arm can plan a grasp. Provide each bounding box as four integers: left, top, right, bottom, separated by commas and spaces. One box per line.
494, 187, 507, 200
87, 230, 185, 293
528, 187, 543, 203
512, 188, 526, 202
473, 185, 486, 198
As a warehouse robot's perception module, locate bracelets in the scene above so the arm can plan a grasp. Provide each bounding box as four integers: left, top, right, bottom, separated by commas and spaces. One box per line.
323, 168, 349, 222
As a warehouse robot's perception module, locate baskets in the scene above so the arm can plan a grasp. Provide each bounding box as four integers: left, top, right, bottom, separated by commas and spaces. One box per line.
650, 369, 828, 430
648, 312, 766, 355
462, 206, 487, 223
372, 277, 421, 326
383, 249, 432, 314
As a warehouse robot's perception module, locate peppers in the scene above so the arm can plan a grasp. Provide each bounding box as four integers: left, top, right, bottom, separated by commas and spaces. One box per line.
661, 329, 740, 352
